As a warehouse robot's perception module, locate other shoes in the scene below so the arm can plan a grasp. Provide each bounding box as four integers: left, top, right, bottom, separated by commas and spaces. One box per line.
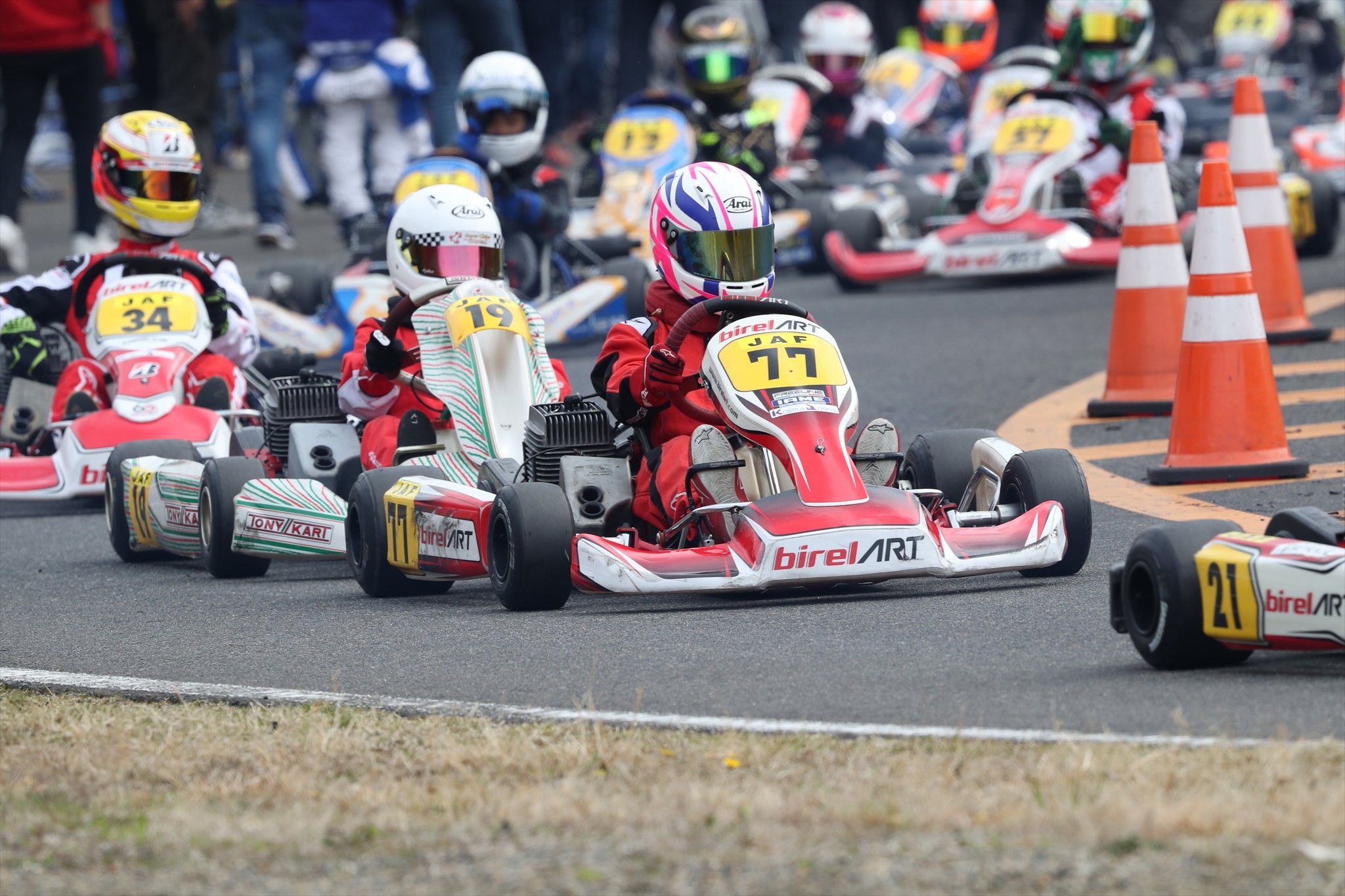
1061, 171, 1098, 240
258, 221, 298, 250
956, 178, 978, 214
192, 375, 231, 426
69, 224, 120, 255
395, 409, 437, 465
196, 198, 260, 237
851, 418, 900, 487
687, 424, 750, 545
61, 391, 99, 435
0, 214, 30, 274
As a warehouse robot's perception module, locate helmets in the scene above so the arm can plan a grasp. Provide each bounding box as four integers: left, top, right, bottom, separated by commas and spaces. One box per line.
650, 161, 776, 316
386, 183, 505, 298
457, 51, 549, 167
91, 110, 203, 237
794, 1, 874, 97
916, 0, 1000, 71
1074, 1, 1154, 80
1045, 0, 1076, 49
681, 5, 759, 85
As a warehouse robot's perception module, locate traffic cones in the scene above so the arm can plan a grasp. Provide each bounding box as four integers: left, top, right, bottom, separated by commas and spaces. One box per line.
1225, 76, 1332, 347
1087, 121, 1193, 421
1147, 161, 1310, 487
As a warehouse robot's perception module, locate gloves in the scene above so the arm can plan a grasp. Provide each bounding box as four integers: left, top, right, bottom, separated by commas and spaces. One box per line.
0, 316, 53, 382
200, 279, 229, 339
505, 190, 542, 226
364, 330, 405, 381
629, 343, 684, 408
1098, 118, 1133, 158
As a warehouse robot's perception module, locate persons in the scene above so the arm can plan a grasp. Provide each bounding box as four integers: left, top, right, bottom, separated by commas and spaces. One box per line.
379, 50, 570, 300
0, 106, 260, 425
591, 160, 900, 547
325, 181, 572, 475
0, 0, 659, 268
648, 0, 1345, 239
